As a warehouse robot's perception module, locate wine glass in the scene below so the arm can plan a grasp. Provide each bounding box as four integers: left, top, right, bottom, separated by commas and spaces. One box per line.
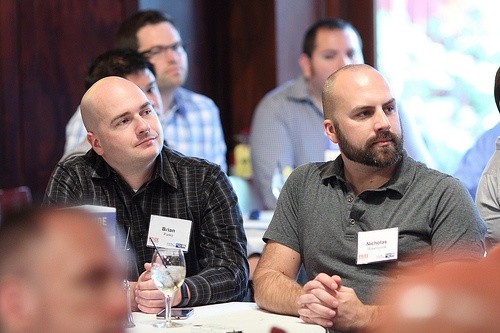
150, 248, 187, 328
272, 165, 295, 199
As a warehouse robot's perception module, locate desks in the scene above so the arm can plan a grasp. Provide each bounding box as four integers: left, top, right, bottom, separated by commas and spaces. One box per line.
127, 302, 327, 333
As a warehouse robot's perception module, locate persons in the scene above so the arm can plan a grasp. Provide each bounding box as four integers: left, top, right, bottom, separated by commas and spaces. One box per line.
254, 64, 488, 333
38, 75, 249, 313
62, 8, 228, 180
249, 17, 420, 210
1, 203, 132, 333
475, 66, 500, 245
453, 122, 499, 203
58, 49, 169, 171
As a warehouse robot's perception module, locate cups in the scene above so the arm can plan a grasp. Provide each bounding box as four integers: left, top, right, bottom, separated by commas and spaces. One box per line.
119, 248, 136, 329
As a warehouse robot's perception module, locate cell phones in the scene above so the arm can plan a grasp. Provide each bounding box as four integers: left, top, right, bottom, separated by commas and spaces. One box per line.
157, 308, 194, 319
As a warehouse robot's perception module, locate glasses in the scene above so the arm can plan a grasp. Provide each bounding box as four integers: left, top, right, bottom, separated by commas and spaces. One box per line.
139, 42, 184, 58
